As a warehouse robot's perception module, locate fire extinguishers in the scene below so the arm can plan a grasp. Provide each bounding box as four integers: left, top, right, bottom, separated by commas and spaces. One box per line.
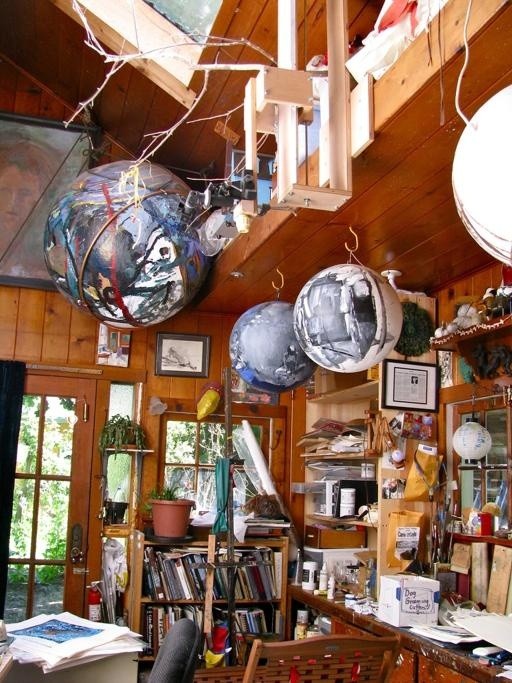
86, 580, 103, 621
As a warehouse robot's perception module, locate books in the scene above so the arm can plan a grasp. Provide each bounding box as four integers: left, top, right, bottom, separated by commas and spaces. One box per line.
469, 541, 489, 607
141, 546, 283, 667
484, 546, 512, 619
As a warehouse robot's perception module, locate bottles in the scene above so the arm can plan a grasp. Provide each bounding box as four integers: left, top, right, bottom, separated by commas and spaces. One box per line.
319, 561, 335, 600
295, 610, 319, 641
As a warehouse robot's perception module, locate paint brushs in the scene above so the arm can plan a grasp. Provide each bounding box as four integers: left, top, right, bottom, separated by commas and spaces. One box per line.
428, 488, 457, 563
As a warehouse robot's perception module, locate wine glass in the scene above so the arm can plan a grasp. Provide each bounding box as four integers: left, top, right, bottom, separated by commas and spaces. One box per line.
334, 565, 347, 595
348, 572, 363, 600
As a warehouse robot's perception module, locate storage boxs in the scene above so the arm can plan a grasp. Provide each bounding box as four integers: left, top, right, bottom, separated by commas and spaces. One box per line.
291, 480, 340, 516
378, 575, 441, 627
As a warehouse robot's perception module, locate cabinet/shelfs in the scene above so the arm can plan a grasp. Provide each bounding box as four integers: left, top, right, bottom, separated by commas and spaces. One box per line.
303, 379, 438, 599
122, 524, 289, 662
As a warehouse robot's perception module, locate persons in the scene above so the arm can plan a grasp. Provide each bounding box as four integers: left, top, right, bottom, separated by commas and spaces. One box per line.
0, 128, 68, 283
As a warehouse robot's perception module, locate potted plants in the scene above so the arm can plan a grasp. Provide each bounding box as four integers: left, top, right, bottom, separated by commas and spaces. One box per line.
134, 482, 195, 538
97, 414, 148, 459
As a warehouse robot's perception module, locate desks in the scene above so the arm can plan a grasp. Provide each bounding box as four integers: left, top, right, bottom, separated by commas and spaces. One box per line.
286, 583, 512, 682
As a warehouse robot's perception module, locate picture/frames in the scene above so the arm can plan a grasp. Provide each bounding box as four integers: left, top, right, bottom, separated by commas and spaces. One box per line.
382, 359, 440, 414
155, 332, 210, 378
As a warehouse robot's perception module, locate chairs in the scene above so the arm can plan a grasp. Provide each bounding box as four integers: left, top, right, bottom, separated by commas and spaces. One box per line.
148, 619, 202, 683
243, 632, 402, 683
